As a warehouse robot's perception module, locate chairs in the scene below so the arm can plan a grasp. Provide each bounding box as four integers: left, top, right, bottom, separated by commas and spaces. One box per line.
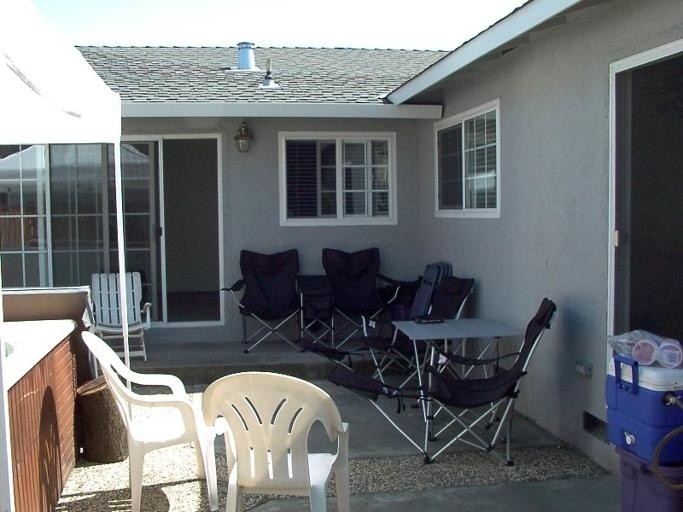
422, 297, 558, 464
201, 371, 351, 511
81, 331, 234, 511
220, 249, 304, 354
82, 270, 150, 361
297, 249, 471, 422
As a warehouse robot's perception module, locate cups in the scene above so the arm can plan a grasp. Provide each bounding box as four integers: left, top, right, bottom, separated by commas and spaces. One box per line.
631, 338, 683, 369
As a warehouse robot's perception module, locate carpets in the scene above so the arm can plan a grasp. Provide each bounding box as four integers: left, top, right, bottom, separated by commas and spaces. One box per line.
53, 442, 604, 511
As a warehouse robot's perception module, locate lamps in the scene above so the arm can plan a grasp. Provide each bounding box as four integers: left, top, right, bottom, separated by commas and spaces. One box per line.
232, 121, 252, 154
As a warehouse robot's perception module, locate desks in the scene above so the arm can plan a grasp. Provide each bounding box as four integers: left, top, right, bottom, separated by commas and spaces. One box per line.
393, 318, 522, 440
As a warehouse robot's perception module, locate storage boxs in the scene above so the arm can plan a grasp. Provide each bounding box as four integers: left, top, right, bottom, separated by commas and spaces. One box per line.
602, 354, 682, 511
